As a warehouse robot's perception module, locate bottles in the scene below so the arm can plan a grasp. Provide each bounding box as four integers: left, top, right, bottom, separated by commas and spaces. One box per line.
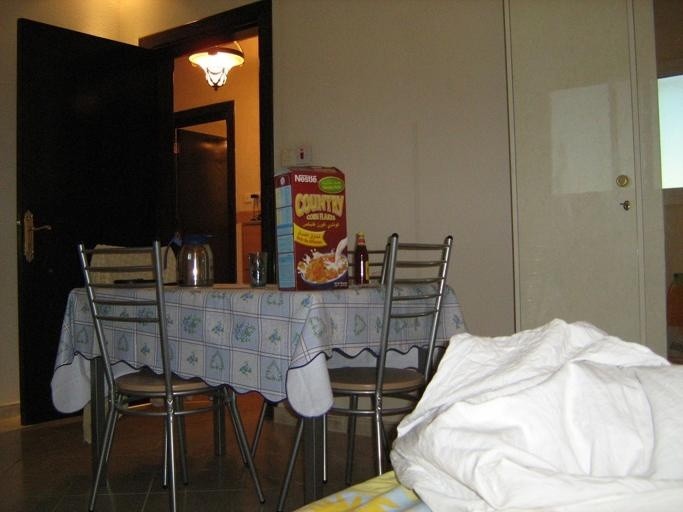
352, 232, 370, 285
250, 193, 259, 221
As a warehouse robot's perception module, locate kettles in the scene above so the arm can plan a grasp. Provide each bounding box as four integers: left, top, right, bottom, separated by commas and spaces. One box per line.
164, 229, 215, 288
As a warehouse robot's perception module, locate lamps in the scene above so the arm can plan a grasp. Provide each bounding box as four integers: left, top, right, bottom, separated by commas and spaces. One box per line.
186, 39, 246, 90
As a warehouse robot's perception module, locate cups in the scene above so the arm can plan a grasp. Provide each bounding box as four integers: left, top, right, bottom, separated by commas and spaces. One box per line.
246, 251, 269, 289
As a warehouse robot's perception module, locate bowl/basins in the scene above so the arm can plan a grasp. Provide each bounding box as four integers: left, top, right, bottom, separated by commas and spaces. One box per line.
300, 253, 349, 289
